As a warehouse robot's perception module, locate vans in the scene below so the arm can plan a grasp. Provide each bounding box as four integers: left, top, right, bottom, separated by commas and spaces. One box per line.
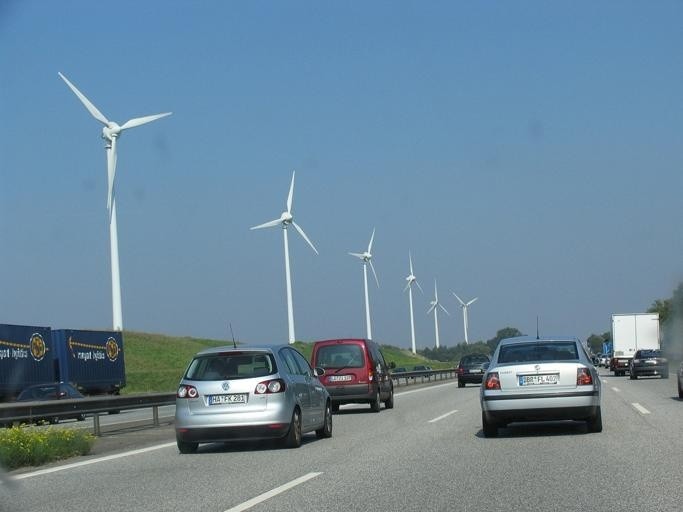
313, 337, 397, 412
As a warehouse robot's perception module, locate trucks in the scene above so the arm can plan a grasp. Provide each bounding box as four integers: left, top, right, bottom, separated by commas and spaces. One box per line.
0, 324, 127, 421
609, 312, 661, 376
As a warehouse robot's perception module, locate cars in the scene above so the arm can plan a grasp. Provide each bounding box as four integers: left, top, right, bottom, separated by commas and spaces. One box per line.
592, 352, 612, 369
390, 365, 434, 380
478, 335, 603, 437
14, 382, 87, 426
457, 351, 492, 389
628, 349, 670, 379
174, 342, 333, 456
